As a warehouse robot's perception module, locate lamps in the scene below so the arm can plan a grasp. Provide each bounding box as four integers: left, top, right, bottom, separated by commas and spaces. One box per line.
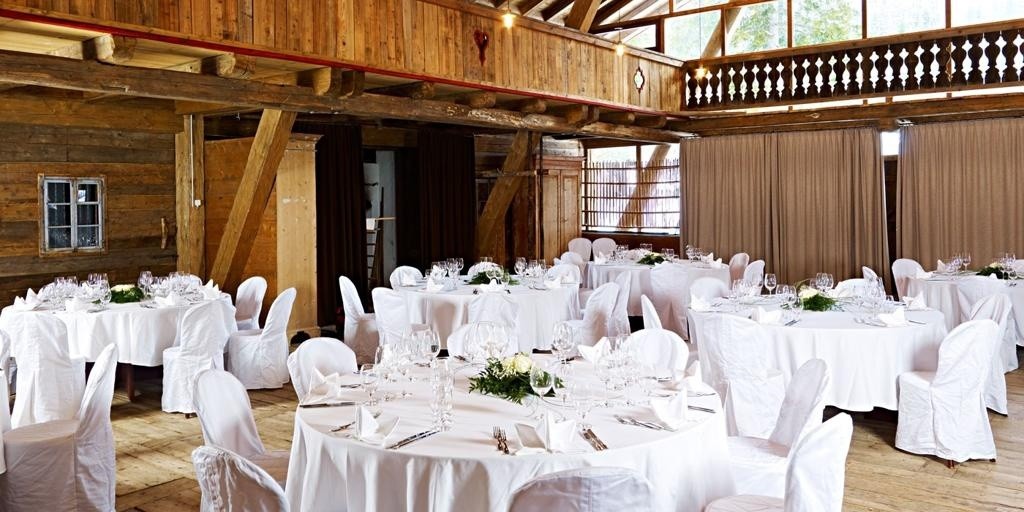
693, 0, 707, 81
501, 0, 518, 28
613, 1, 626, 55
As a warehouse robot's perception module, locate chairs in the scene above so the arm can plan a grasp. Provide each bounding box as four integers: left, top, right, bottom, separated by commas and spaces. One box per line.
4, 343, 118, 512
193, 445, 286, 512
193, 370, 292, 484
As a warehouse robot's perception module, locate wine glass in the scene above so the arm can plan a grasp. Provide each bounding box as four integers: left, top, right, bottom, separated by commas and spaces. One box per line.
359, 322, 634, 433
945, 253, 1017, 285
54, 271, 201, 310
426, 257, 546, 291
732, 273, 894, 321
609, 243, 702, 264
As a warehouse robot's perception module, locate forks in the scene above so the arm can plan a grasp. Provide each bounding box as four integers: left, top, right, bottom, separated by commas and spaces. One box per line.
493, 426, 503, 450
501, 430, 509, 454
